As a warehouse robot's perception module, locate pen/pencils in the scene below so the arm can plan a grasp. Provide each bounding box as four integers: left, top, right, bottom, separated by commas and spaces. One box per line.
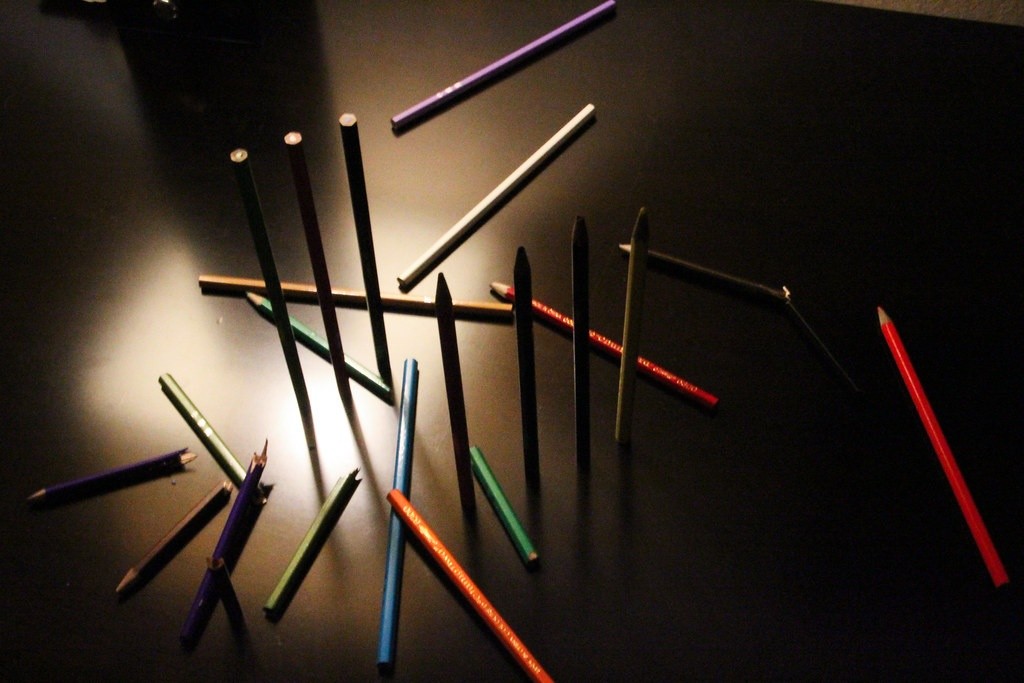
20, 111, 794, 682
872, 302, 1008, 589
382, 487, 552, 682
483, 278, 721, 412
392, 1, 622, 135
400, 103, 601, 288
